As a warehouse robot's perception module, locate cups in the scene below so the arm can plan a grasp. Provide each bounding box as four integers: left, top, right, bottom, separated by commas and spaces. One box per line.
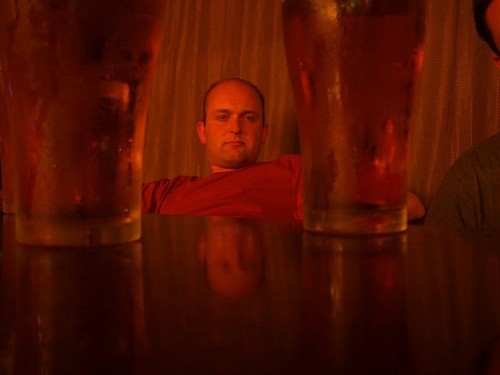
282, 0, 428, 235
0, 0, 173, 247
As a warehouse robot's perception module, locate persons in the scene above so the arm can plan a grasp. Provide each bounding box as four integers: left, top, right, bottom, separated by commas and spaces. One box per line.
197, 215, 266, 306
139, 78, 426, 222
430, 0, 500, 227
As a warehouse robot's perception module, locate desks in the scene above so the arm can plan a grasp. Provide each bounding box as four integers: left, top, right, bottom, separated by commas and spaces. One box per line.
0, 213, 500, 375
